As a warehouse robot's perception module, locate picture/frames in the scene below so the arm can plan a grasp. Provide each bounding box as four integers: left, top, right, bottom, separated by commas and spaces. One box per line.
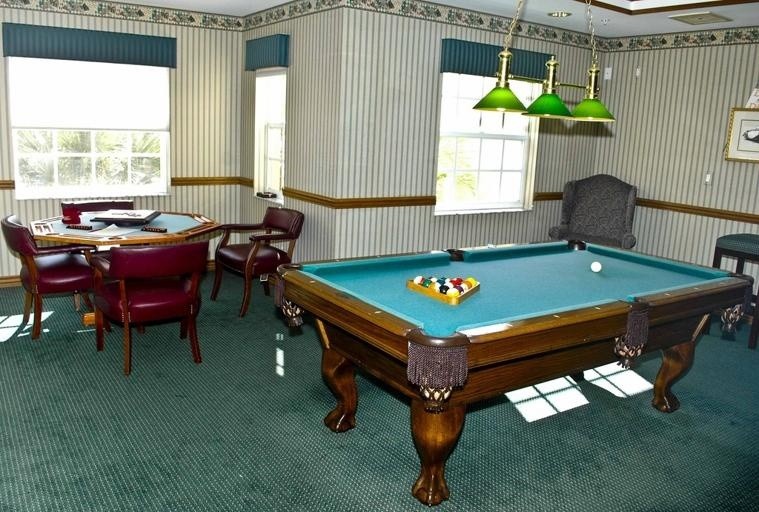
725, 108, 759, 163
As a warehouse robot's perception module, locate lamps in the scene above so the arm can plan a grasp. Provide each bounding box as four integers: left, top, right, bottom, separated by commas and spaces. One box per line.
472, 0, 616, 123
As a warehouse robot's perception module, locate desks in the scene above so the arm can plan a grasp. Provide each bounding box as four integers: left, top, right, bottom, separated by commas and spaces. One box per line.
276, 238, 754, 507
28, 210, 221, 332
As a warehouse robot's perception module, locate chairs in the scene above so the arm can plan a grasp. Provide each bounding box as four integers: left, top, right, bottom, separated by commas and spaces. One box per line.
60, 199, 136, 218
0, 214, 112, 340
210, 206, 305, 318
548, 174, 637, 250
86, 239, 210, 376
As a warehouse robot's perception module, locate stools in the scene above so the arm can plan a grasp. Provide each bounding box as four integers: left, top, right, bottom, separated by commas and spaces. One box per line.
703, 233, 759, 349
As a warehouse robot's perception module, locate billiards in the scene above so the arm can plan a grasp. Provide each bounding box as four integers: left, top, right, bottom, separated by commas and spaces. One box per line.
414, 275, 477, 298
592, 261, 602, 274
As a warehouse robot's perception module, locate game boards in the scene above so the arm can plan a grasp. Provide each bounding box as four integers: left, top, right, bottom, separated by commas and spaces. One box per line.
90, 209, 161, 224
